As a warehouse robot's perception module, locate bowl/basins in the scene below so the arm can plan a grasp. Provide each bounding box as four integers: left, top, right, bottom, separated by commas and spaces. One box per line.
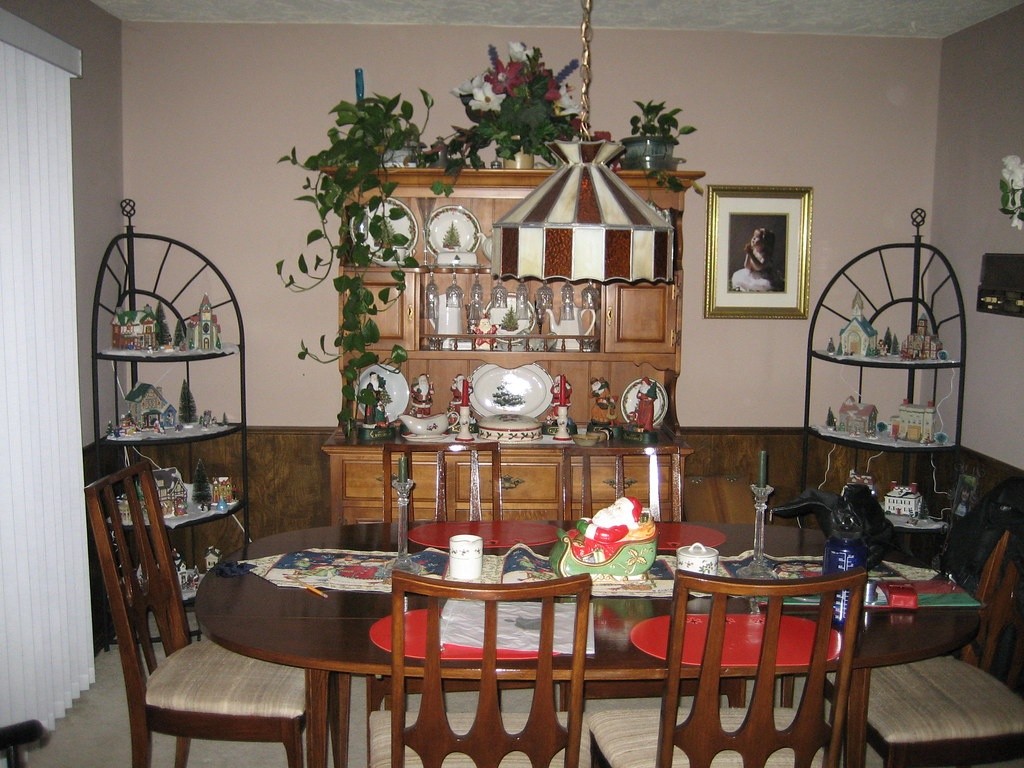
587, 432, 605, 442
572, 434, 599, 447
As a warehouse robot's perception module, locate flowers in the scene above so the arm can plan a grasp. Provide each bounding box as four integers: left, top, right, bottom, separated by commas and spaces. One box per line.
465, 42, 577, 131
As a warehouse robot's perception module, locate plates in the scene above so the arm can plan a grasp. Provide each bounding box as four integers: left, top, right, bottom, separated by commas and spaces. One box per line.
401, 432, 447, 441
468, 362, 554, 417
349, 197, 418, 262
620, 378, 669, 426
425, 204, 481, 257
353, 364, 410, 423
485, 292, 536, 342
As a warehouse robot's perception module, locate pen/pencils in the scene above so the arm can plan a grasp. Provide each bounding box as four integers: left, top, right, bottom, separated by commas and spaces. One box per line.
287, 575, 328, 598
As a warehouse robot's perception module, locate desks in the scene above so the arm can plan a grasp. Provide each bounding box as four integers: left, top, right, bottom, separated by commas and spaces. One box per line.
192, 522, 983, 768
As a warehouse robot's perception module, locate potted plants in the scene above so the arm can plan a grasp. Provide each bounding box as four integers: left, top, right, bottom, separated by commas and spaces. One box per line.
623, 99, 693, 174
271, 97, 414, 445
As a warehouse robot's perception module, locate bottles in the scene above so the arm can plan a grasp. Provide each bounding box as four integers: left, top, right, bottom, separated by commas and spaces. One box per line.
822, 530, 866, 631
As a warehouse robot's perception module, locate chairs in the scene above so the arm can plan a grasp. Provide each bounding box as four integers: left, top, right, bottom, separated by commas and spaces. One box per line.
382, 441, 503, 525
391, 573, 590, 768
866, 527, 1024, 767
560, 446, 682, 524
85, 462, 303, 768
657, 567, 865, 768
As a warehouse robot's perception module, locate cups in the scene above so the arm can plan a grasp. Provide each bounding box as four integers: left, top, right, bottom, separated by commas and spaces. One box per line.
676, 543, 719, 594
449, 534, 484, 580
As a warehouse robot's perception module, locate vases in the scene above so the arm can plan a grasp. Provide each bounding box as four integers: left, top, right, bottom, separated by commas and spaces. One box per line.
497, 135, 539, 171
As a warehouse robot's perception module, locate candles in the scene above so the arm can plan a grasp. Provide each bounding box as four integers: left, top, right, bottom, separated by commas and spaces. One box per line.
461, 379, 469, 404
758, 447, 770, 487
398, 451, 408, 483
559, 374, 567, 404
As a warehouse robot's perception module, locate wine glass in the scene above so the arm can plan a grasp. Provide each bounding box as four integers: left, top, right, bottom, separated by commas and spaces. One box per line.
426, 263, 598, 320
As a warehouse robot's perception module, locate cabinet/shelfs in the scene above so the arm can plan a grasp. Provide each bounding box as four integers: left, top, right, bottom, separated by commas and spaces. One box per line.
323, 170, 708, 559
93, 198, 252, 655
803, 209, 967, 540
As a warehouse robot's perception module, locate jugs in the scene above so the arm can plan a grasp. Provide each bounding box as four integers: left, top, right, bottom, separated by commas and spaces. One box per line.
425, 290, 465, 348
399, 411, 460, 436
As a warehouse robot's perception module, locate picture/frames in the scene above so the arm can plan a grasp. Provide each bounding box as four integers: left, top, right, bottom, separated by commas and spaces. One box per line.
706, 184, 812, 320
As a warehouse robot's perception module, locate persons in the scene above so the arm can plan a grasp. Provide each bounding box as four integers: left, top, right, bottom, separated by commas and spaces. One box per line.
450, 374, 472, 413
364, 372, 387, 427
731, 228, 778, 294
588, 377, 620, 427
627, 377, 656, 432
412, 373, 435, 418
561, 496, 642, 563
550, 374, 572, 416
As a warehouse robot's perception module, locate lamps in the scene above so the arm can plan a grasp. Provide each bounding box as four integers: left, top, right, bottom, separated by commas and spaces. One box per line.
491, 1, 673, 286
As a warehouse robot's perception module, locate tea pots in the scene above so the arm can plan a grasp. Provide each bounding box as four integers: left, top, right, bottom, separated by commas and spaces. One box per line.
476, 231, 492, 262
545, 302, 596, 350
